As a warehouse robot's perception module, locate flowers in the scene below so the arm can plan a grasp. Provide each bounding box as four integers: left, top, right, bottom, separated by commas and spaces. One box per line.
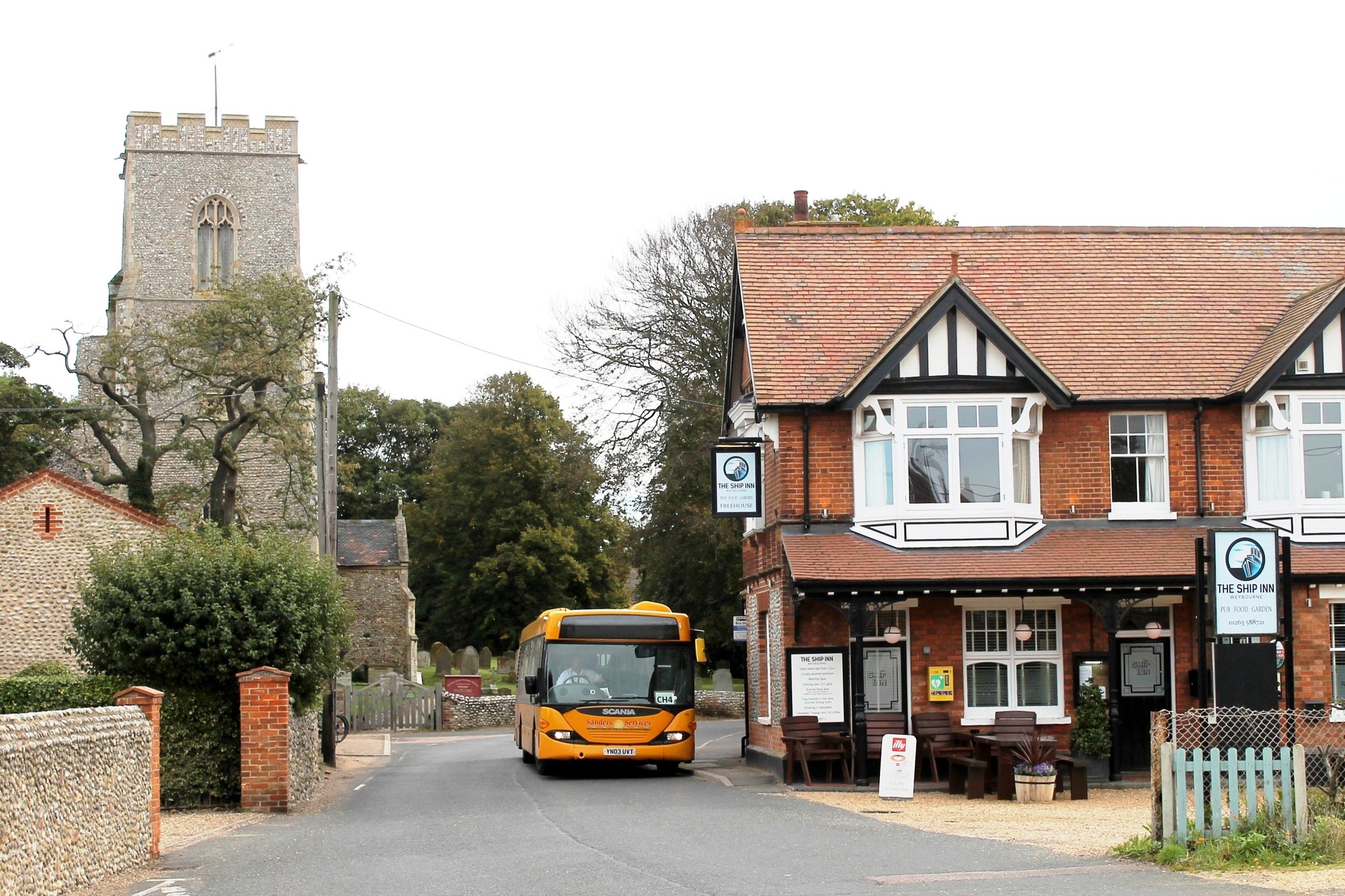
1004, 727, 1065, 776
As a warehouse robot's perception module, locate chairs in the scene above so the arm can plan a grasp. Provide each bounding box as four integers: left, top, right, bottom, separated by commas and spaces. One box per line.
911, 712, 974, 784
779, 716, 852, 786
850, 713, 906, 784
991, 711, 1036, 757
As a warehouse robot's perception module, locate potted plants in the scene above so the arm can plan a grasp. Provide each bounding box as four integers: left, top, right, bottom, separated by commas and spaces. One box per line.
1066, 677, 1113, 783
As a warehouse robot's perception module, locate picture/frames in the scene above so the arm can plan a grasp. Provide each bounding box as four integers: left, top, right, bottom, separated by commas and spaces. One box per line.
785, 645, 851, 732
710, 447, 762, 518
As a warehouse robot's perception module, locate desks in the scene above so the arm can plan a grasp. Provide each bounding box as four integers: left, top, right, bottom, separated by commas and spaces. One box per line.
974, 735, 1059, 800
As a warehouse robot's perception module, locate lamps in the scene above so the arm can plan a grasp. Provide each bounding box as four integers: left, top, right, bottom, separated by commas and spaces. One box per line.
1144, 597, 1162, 639
1013, 590, 1033, 641
883, 602, 902, 644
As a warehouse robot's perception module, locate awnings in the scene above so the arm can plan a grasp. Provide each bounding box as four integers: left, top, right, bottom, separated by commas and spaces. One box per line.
778, 527, 1344, 603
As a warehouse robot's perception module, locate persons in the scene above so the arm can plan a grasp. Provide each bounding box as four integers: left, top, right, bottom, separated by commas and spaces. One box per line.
555, 655, 607, 684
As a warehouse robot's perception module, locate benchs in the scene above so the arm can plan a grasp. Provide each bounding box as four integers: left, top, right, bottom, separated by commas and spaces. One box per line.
1056, 754, 1088, 800
948, 756, 987, 800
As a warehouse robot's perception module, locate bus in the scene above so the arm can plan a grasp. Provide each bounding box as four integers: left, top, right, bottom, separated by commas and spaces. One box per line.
513, 601, 709, 777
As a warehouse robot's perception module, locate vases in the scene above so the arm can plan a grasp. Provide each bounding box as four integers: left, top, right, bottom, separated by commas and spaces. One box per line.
1014, 775, 1056, 801
